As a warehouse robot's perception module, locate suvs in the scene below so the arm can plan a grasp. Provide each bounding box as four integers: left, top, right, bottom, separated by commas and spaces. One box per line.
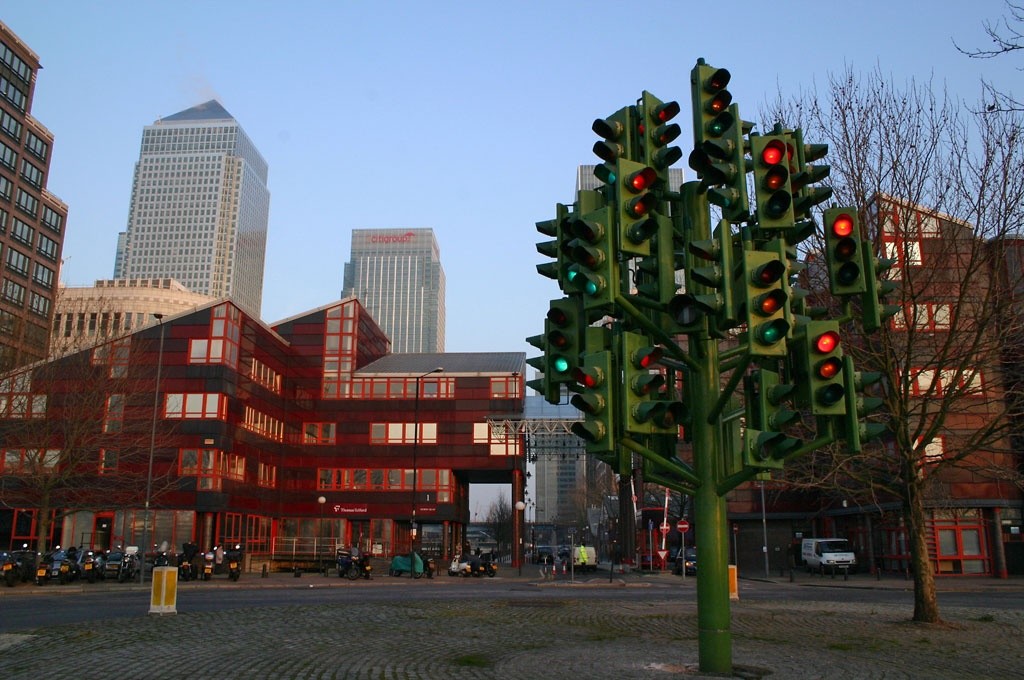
533, 547, 554, 564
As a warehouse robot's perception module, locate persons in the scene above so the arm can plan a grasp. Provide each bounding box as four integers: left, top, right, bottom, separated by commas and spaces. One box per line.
214, 544, 223, 576
579, 541, 589, 575
455, 541, 495, 563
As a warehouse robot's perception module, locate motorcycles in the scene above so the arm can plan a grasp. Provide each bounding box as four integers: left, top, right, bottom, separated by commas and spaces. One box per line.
392, 552, 423, 577
448, 553, 497, 577
36, 544, 62, 584
0, 542, 34, 586
338, 547, 365, 579
223, 544, 243, 581
201, 546, 218, 581
65, 541, 198, 583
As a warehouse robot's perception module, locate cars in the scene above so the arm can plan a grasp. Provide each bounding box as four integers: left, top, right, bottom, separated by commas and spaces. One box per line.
558, 547, 570, 559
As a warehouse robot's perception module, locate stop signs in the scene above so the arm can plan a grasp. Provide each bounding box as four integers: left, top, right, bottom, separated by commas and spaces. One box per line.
660, 522, 670, 534
733, 524, 738, 534
676, 521, 690, 533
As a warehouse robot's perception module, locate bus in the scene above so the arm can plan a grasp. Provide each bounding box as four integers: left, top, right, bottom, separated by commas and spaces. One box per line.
608, 507, 668, 570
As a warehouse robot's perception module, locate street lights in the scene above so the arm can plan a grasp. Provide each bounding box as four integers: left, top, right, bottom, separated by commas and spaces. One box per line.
754, 472, 771, 579
318, 496, 327, 573
516, 501, 526, 576
139, 312, 163, 579
411, 368, 445, 548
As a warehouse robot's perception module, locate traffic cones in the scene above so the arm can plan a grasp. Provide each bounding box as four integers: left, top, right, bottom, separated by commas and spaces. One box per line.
552, 562, 557, 575
544, 563, 548, 574
619, 561, 625, 573
562, 561, 567, 575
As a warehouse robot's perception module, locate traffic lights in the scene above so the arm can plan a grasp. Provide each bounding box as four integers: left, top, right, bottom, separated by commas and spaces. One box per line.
526, 63, 906, 468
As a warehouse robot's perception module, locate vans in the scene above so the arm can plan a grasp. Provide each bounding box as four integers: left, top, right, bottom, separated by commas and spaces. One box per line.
48, 548, 92, 579
571, 546, 598, 571
801, 538, 856, 574
